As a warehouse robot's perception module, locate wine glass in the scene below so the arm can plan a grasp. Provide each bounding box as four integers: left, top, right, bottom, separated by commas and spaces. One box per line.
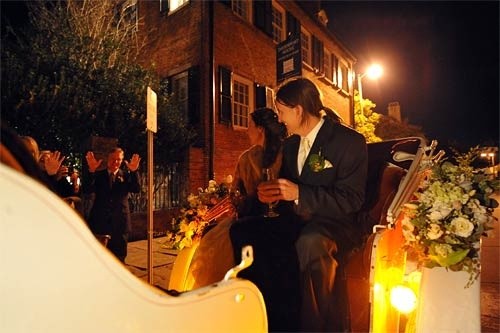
261, 167, 280, 218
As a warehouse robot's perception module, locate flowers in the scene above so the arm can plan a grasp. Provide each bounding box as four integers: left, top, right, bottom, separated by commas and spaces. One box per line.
309, 152, 333, 172
395, 144, 500, 291
164, 170, 232, 250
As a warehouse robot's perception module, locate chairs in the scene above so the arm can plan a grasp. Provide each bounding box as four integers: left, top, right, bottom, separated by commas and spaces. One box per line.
0, 163, 269, 333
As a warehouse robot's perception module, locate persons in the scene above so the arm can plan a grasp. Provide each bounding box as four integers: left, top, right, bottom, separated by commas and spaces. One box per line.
21, 135, 74, 197
257, 77, 370, 333
230, 108, 302, 333
79, 147, 141, 260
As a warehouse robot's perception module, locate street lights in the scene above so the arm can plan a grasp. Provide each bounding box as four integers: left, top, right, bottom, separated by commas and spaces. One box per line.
356, 64, 381, 129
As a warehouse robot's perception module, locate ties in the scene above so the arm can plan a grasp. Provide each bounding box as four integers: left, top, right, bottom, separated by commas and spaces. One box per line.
297, 137, 310, 175
109, 172, 116, 189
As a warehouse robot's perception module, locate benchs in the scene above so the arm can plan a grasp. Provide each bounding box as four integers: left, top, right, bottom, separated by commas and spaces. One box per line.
167, 137, 425, 283
